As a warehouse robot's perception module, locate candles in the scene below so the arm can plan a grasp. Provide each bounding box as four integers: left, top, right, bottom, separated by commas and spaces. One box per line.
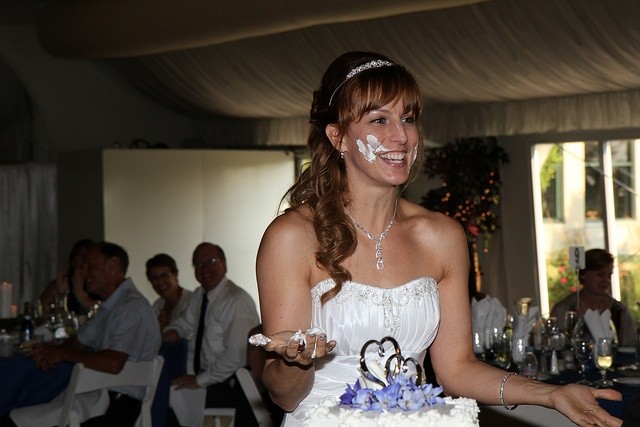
0, 281, 13, 319
10, 303, 16, 318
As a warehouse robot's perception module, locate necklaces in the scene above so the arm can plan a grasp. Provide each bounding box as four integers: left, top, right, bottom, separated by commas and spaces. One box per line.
337, 198, 401, 270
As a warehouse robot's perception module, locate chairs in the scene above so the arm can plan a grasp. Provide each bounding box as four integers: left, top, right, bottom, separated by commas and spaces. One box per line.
44, 355, 165, 427
199, 407, 235, 427
235, 368, 274, 427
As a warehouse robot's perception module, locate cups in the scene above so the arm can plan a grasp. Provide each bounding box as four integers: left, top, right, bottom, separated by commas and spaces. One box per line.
545, 317, 558, 338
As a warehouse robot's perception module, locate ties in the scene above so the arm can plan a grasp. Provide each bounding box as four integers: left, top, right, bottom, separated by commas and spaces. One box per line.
194, 293, 208, 375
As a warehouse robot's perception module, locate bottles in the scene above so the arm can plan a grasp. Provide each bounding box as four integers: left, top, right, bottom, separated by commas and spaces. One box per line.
20, 301, 34, 343
549, 348, 560, 375
539, 347, 548, 373
520, 345, 538, 381
512, 297, 531, 364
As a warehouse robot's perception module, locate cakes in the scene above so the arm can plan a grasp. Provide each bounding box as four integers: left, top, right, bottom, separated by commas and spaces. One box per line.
304, 369, 481, 426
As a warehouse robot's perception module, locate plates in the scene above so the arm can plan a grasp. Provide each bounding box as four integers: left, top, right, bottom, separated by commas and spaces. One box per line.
612, 377, 640, 388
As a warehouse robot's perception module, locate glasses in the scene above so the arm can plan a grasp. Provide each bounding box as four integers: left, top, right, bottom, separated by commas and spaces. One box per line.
196, 258, 220, 266
150, 272, 172, 281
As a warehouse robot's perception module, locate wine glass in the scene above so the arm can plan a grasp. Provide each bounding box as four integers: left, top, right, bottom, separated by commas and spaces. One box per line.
488, 332, 510, 372
564, 311, 577, 351
569, 338, 595, 387
595, 337, 614, 387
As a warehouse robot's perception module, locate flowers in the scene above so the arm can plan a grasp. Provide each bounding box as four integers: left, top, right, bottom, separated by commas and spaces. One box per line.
340, 368, 446, 413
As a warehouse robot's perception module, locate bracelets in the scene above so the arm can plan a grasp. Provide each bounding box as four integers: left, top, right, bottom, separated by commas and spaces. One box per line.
499, 371, 522, 414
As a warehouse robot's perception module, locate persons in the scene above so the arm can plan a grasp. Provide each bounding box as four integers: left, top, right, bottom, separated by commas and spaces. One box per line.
29, 242, 161, 427
40, 241, 99, 316
549, 249, 639, 362
144, 252, 195, 358
249, 51, 625, 425
165, 242, 263, 424
